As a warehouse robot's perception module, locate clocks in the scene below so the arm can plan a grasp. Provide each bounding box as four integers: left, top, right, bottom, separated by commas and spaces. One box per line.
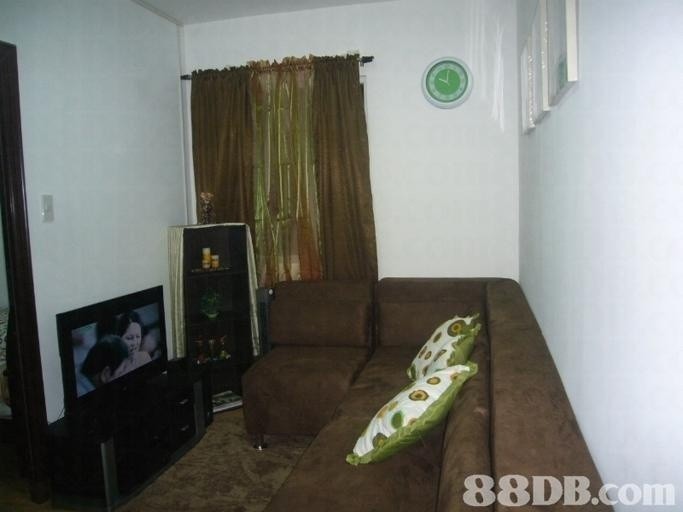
420, 55, 474, 110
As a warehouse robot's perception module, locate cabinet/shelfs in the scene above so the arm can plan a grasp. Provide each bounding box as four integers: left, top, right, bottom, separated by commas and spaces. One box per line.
169, 222, 257, 415
40, 357, 221, 510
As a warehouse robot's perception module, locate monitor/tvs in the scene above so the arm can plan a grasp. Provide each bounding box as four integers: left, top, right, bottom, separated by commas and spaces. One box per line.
57, 284, 168, 419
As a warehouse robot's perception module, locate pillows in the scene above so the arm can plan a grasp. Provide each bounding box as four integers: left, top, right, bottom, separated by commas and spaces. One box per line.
346, 310, 483, 469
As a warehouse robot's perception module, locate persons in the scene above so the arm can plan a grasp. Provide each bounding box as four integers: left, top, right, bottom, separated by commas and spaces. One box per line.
112, 311, 153, 378
75, 335, 128, 398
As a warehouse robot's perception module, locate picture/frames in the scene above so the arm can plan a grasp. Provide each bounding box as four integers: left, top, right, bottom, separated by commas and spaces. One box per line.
520, 0, 580, 137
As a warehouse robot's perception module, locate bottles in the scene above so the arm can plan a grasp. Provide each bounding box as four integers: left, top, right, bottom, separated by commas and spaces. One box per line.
202, 246, 220, 272
196, 340, 229, 363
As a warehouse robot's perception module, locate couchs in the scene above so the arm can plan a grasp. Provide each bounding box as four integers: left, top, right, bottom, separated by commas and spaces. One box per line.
239, 277, 606, 510
